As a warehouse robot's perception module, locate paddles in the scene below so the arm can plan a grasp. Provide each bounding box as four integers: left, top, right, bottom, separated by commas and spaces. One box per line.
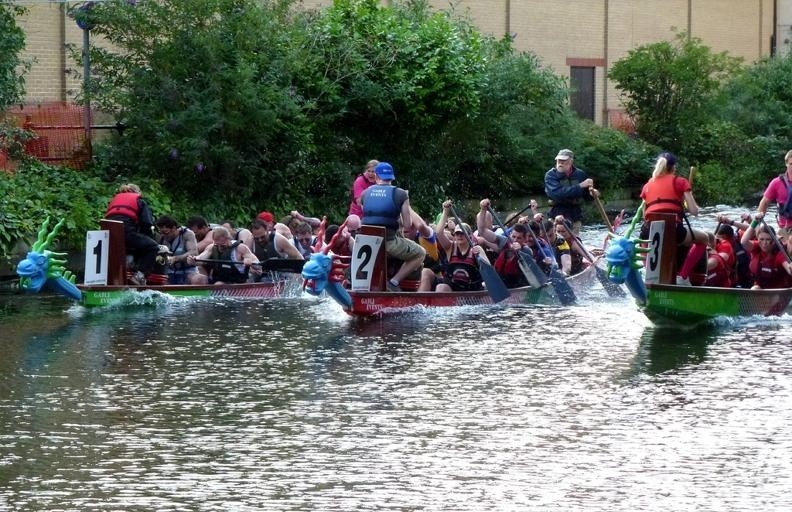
193, 258, 309, 273
449, 203, 627, 307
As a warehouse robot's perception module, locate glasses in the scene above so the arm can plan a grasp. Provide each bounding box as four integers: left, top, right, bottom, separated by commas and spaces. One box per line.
299, 237, 312, 243
213, 241, 227, 247
254, 233, 266, 239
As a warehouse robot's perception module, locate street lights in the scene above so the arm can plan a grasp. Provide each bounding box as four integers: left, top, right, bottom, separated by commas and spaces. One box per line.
74, 7, 97, 159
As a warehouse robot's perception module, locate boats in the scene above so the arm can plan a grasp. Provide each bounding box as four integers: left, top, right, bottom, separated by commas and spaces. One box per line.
299, 209, 628, 319
16, 213, 309, 308
603, 232, 791, 334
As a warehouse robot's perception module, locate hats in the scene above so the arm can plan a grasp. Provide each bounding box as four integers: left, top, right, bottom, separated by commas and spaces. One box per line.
555, 149, 573, 162
453, 223, 470, 234
256, 211, 272, 223
373, 162, 395, 180
658, 153, 678, 163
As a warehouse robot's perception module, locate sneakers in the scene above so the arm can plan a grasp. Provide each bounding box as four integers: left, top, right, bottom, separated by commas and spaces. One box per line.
676, 275, 692, 285
386, 279, 402, 292
131, 273, 146, 286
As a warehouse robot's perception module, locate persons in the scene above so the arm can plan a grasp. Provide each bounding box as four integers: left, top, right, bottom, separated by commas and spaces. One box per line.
341, 160, 426, 290
639, 153, 709, 286
690, 211, 792, 290
758, 150, 792, 242
544, 149, 599, 235
148, 211, 339, 285
102, 184, 159, 286
401, 198, 583, 291
